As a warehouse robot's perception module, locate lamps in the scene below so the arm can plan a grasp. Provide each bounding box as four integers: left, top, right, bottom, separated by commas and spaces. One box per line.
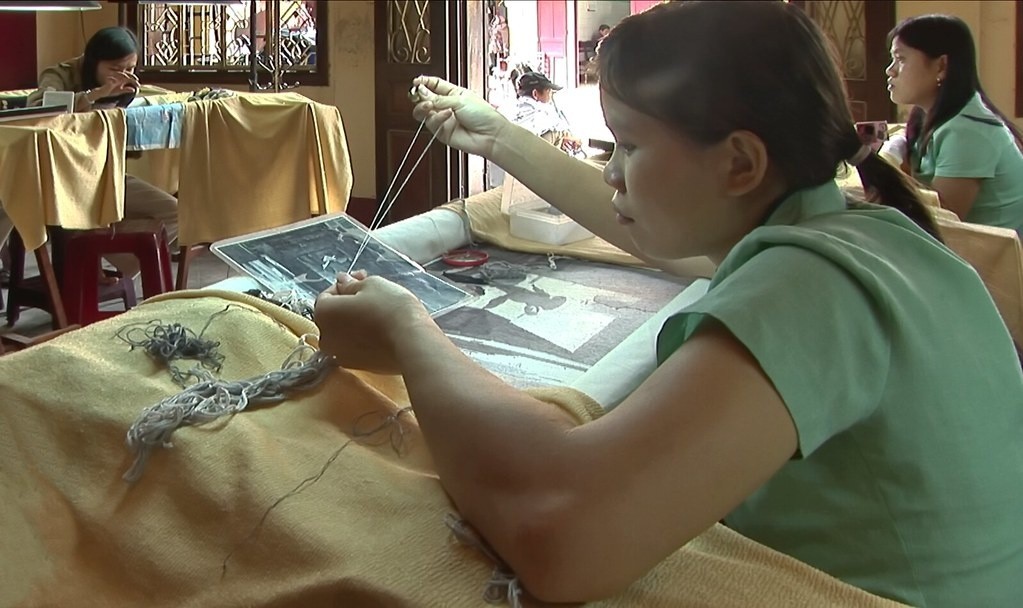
0, 0, 103, 12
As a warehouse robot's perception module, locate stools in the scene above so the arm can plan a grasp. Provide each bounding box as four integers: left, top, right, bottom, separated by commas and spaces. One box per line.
57, 216, 175, 334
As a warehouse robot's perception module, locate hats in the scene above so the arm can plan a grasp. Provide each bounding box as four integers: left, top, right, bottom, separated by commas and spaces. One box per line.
518, 73, 563, 96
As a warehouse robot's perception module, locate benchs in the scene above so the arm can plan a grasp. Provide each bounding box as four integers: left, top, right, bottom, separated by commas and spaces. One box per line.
579, 41, 600, 83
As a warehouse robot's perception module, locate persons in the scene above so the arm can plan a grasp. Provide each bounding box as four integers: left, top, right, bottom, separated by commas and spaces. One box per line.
506, 26, 612, 162
29, 25, 183, 288
315, 1, 1023, 608
886, 16, 1023, 240
